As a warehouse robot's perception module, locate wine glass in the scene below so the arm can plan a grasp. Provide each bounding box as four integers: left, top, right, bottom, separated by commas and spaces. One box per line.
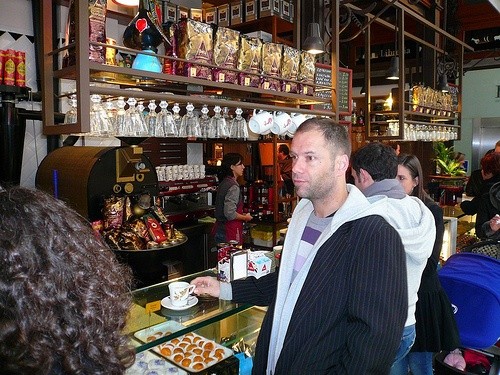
63, 90, 250, 139
387, 121, 458, 141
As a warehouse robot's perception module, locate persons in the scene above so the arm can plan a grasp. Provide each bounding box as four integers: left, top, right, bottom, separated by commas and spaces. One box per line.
190, 117, 408, 375
277, 145, 294, 198
388, 153, 459, 375
351, 141, 436, 369
390, 143, 400, 155
457, 140, 500, 239
214, 153, 253, 248
0, 185, 134, 375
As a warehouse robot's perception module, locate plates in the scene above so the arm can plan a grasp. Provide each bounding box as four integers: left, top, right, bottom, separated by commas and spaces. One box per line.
160, 296, 199, 310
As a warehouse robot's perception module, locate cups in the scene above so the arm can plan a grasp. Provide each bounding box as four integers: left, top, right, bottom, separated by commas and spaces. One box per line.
248, 109, 274, 135
269, 110, 293, 134
290, 113, 316, 135
155, 164, 206, 181
272, 245, 282, 271
168, 281, 196, 307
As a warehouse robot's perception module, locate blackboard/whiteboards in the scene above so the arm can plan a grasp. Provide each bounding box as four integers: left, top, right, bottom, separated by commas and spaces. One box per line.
311, 62, 352, 115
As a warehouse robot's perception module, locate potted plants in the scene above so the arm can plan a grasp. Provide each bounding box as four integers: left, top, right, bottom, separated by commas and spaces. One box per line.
426, 140, 467, 192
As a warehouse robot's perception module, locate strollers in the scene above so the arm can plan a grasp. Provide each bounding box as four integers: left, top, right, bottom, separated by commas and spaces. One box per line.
436, 238, 500, 375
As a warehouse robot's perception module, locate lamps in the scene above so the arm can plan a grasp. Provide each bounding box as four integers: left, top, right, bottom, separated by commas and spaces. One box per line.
302, 0, 326, 54
438, 0, 451, 92
385, 8, 400, 79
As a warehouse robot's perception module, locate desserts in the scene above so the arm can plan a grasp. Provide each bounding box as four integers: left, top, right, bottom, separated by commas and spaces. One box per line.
148, 330, 225, 371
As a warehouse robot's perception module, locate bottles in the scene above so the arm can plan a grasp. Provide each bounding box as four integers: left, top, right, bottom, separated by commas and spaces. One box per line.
105, 37, 119, 67
242, 185, 291, 221
351, 98, 402, 136
